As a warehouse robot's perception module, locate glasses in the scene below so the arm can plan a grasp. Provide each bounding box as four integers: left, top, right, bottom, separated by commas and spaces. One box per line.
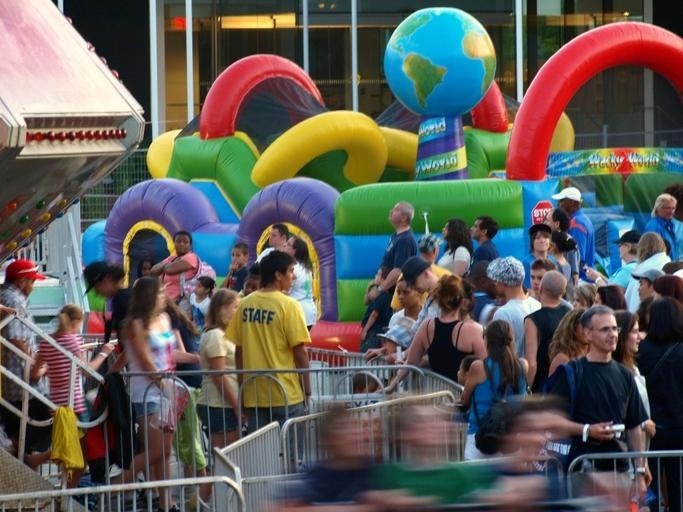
17, 264, 39, 274
589, 327, 622, 334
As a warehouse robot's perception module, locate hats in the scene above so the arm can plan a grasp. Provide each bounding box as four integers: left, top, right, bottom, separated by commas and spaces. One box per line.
630, 269, 663, 284
376, 325, 413, 349
417, 232, 445, 253
612, 230, 641, 245
551, 186, 582, 202
528, 222, 551, 236
485, 255, 526, 287
4, 259, 46, 280
82, 260, 110, 295
400, 255, 433, 289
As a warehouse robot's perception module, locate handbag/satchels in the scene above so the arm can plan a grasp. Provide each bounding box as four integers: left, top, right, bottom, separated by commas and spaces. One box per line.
526, 363, 576, 475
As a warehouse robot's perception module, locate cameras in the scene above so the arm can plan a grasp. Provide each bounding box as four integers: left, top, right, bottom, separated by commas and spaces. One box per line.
605, 423, 625, 438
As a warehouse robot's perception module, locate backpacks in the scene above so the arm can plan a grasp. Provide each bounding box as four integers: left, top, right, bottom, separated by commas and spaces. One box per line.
472, 359, 516, 455
178, 252, 216, 301
